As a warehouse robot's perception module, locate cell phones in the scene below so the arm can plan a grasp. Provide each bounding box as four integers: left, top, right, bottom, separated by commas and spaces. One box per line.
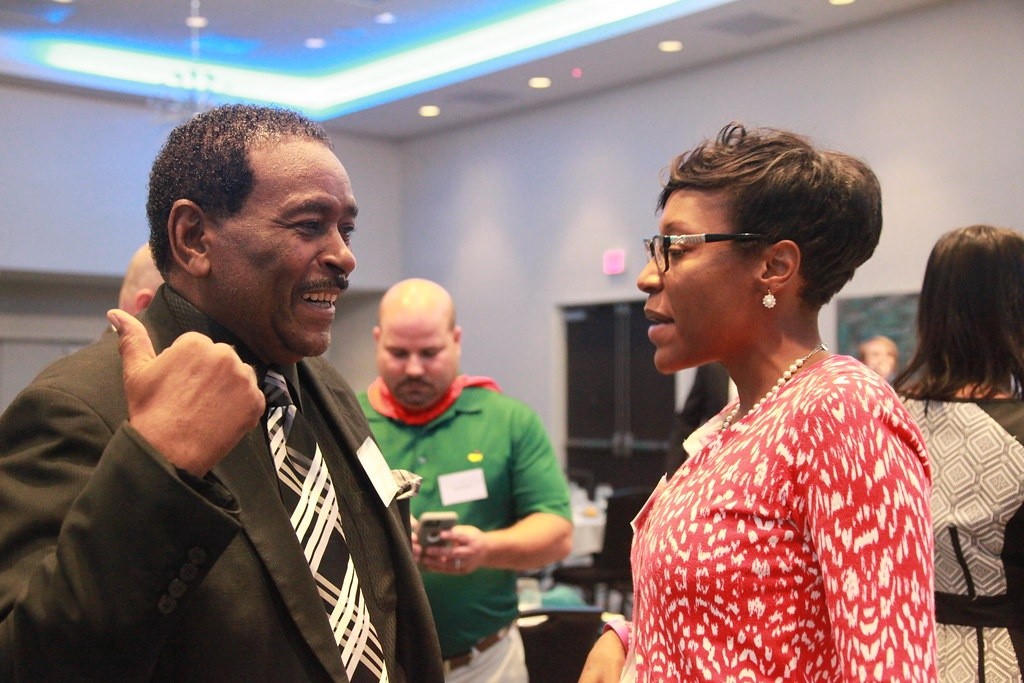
415, 510, 458, 572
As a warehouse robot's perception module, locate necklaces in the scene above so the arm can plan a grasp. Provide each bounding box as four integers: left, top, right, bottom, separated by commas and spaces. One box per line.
720, 341, 829, 433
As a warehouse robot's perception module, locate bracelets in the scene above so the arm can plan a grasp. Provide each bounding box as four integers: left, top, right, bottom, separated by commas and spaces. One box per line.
602, 619, 629, 658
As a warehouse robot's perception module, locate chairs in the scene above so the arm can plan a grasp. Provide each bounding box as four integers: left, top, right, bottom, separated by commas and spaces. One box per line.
552, 498, 641, 606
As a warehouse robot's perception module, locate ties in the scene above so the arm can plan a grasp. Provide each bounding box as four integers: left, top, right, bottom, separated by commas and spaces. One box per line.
263, 368, 390, 683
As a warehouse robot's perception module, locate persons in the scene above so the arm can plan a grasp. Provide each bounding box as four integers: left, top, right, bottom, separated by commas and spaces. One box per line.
575, 121, 938, 682
354, 276, 576, 683
893, 224, 1024, 681
0, 104, 447, 683
101, 241, 165, 342
858, 332, 900, 384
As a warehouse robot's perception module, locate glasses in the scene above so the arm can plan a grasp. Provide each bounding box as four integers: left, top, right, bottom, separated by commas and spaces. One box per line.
643, 232, 775, 274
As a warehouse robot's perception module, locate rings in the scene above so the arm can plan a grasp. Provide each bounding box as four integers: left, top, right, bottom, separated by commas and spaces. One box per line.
456, 559, 461, 570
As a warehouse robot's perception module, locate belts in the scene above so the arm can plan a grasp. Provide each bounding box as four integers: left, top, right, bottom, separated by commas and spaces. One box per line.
443, 624, 511, 670
